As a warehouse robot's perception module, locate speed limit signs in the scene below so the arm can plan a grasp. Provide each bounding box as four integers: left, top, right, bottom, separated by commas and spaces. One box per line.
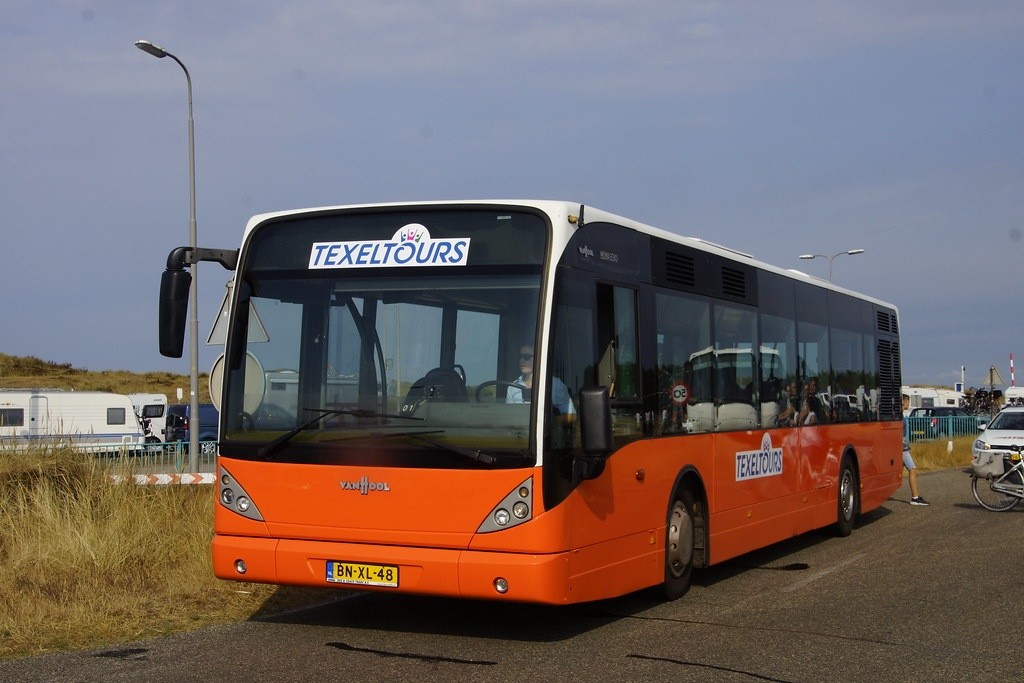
672, 385, 687, 403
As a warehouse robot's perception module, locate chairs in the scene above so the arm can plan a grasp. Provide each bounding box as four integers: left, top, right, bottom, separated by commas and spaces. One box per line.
425, 367, 469, 403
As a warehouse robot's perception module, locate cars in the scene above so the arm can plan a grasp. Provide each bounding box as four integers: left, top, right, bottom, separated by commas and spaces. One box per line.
815, 392, 858, 408
909, 407, 969, 440
970, 405, 1024, 470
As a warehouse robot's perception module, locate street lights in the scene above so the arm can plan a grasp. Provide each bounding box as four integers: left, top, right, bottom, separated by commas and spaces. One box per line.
134, 36, 203, 473
798, 249, 863, 282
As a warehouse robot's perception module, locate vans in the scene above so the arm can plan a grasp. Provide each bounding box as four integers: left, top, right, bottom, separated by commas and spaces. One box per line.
164, 403, 298, 454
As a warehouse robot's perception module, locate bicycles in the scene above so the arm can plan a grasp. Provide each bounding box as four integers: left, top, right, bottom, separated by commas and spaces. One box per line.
972, 444, 1024, 512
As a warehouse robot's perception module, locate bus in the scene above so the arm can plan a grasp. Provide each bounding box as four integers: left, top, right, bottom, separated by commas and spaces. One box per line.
684, 343, 789, 435
157, 197, 906, 620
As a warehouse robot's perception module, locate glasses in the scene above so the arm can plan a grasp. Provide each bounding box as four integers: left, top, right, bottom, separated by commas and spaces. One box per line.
518, 353, 534, 361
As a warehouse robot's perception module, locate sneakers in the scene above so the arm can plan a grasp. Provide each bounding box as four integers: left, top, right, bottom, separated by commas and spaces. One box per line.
910, 496, 931, 506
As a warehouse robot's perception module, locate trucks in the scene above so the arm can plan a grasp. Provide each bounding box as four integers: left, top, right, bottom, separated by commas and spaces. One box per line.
0, 386, 167, 455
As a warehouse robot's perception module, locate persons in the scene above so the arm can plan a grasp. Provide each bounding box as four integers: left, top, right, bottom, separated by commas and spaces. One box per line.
902, 394, 930, 505
774, 376, 820, 427
610, 403, 669, 432
507, 339, 576, 422
